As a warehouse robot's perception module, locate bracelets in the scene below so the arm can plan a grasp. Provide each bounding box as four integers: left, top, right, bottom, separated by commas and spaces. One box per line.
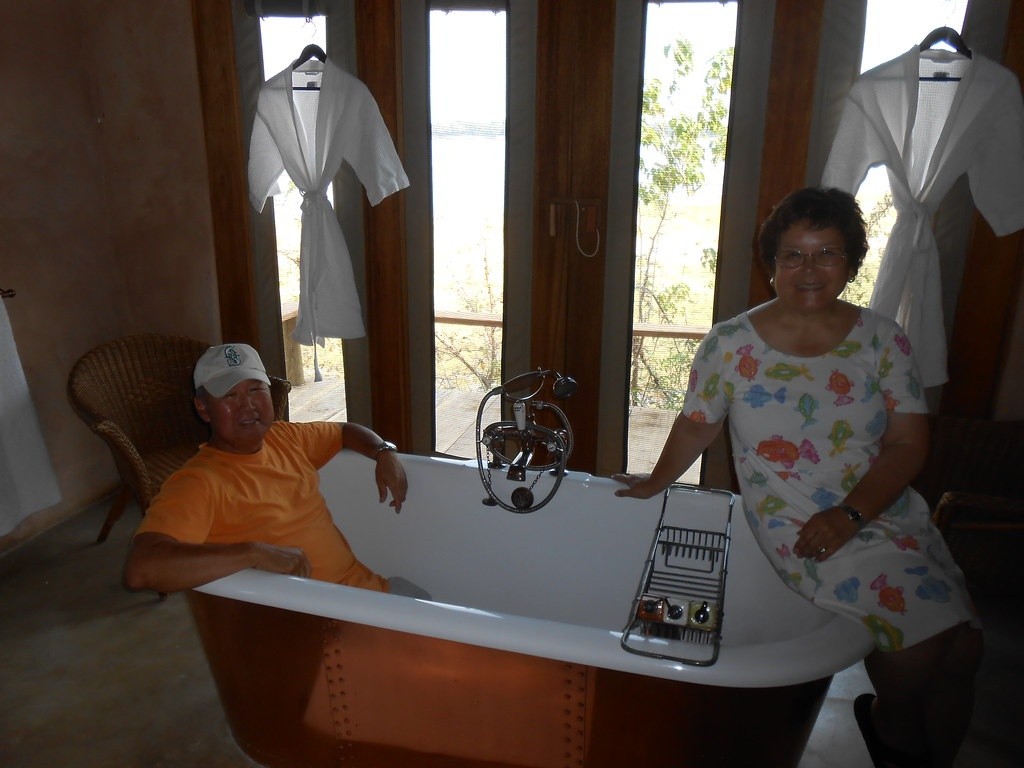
838, 504, 865, 527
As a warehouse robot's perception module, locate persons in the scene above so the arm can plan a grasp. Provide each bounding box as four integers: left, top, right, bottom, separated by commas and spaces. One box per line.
610, 185, 982, 768
122, 343, 430, 600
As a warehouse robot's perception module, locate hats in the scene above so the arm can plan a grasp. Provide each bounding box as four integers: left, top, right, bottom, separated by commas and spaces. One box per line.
193, 344, 271, 397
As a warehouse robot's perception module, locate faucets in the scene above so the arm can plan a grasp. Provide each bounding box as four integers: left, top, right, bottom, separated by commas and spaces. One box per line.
504, 441, 536, 482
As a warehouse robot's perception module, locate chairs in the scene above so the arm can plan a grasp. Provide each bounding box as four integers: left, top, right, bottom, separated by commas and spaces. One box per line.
68, 332, 292, 600
895, 415, 1023, 594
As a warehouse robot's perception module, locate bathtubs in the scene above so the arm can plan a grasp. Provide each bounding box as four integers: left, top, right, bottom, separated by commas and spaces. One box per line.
184, 447, 878, 768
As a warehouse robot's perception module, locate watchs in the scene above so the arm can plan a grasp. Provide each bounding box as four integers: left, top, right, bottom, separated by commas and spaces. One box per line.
375, 441, 397, 454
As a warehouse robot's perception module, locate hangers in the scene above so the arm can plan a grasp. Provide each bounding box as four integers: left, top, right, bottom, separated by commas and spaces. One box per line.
263, 18, 366, 93
858, 22, 1013, 87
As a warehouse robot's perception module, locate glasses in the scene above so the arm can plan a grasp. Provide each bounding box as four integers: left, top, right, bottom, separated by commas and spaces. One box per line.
773, 247, 850, 269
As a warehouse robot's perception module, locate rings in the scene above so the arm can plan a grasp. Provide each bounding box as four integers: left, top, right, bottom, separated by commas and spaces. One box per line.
816, 545, 826, 552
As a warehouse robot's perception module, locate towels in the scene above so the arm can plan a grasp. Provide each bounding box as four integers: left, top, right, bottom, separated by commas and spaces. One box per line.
0, 293, 63, 539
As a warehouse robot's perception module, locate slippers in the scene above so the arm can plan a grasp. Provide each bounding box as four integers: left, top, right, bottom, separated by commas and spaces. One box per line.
854, 694, 918, 768
